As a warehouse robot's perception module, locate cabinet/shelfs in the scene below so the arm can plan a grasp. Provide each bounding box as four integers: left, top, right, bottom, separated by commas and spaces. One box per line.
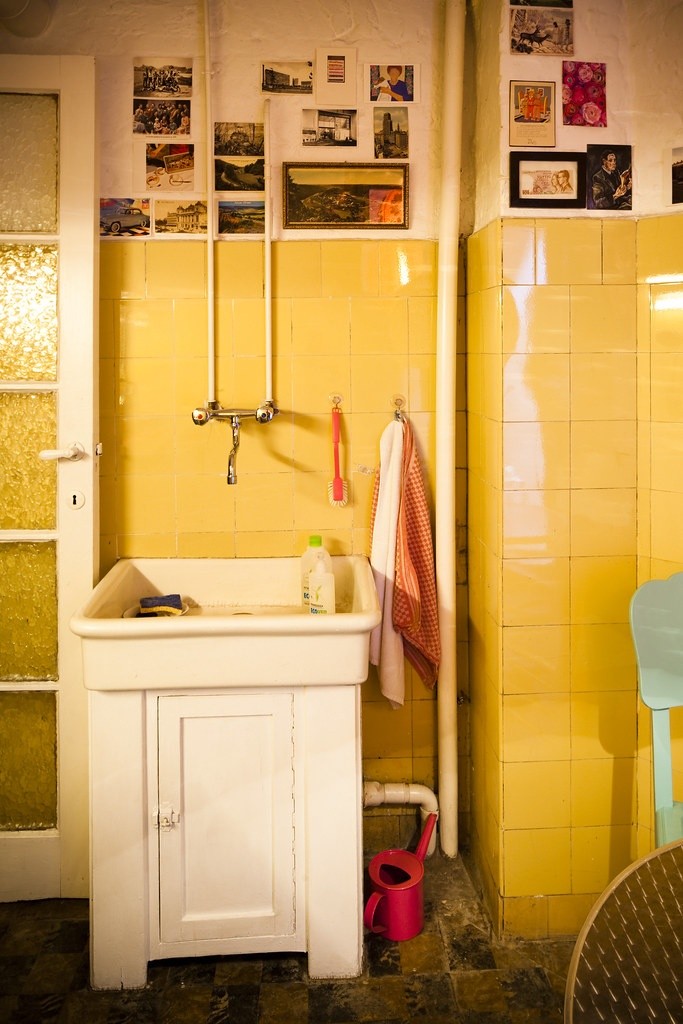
87, 685, 365, 991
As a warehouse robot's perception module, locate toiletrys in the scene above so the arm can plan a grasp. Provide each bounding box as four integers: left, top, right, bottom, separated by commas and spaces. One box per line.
299, 535, 335, 613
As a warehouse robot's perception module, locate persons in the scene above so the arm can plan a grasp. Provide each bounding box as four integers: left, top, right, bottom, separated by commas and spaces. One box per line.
375, 66, 409, 101
133, 101, 189, 135
141, 64, 180, 91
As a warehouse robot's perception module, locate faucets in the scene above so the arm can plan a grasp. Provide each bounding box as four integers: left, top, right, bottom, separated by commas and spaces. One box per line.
227, 416, 241, 484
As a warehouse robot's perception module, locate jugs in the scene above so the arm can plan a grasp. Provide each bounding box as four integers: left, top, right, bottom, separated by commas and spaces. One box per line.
364, 814, 436, 941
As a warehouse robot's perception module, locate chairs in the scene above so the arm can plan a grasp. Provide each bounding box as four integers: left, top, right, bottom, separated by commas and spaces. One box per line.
629, 572, 683, 847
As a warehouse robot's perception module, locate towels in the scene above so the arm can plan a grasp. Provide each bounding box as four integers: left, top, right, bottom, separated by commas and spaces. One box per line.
369, 418, 442, 708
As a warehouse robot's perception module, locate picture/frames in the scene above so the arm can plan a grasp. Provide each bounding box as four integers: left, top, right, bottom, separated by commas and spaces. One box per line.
509, 151, 586, 208
282, 162, 410, 229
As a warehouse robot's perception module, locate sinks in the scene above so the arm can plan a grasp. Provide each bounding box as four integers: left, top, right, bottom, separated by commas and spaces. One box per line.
68, 555, 382, 692
